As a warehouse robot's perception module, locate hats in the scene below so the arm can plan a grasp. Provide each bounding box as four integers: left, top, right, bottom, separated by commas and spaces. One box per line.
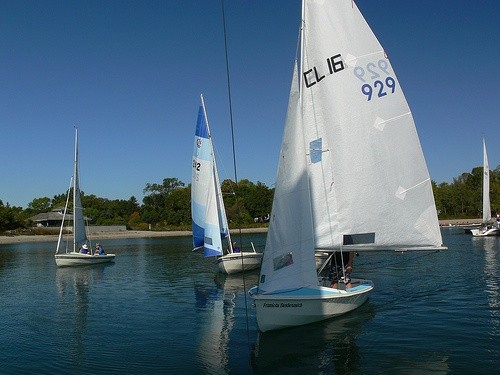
82, 244, 88, 249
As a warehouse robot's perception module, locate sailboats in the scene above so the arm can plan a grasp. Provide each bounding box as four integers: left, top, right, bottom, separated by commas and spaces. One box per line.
190, 91, 264, 275
247, 0, 448, 336
463, 137, 500, 238
53, 122, 115, 268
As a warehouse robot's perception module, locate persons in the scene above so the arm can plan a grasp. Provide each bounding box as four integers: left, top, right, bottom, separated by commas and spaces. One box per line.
82, 244, 88, 254
233, 242, 240, 253
328, 250, 353, 289
96, 243, 103, 254
